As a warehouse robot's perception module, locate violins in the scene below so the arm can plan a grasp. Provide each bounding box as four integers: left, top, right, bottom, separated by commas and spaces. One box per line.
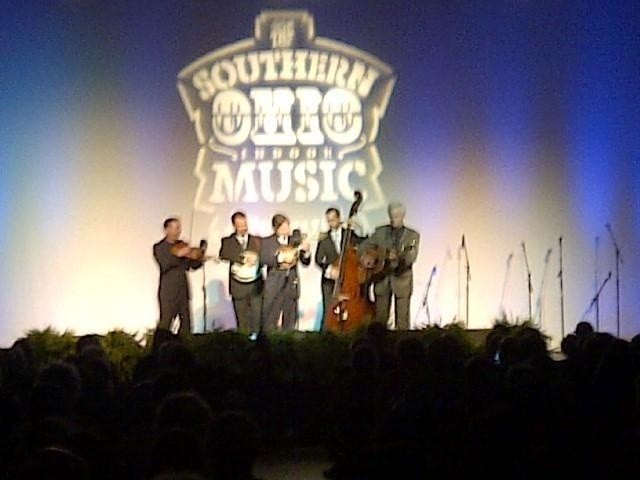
171, 241, 221, 267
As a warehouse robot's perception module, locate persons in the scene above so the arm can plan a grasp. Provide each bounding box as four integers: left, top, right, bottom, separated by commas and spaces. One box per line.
260, 212, 311, 332
354, 200, 420, 332
315, 207, 368, 333
0, 320, 640, 480
153, 218, 209, 335
219, 210, 264, 336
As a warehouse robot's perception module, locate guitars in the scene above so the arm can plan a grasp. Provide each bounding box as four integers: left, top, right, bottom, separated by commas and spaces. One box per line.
357, 238, 413, 284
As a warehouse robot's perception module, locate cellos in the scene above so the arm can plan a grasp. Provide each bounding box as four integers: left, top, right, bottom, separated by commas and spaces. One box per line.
325, 190, 376, 334
